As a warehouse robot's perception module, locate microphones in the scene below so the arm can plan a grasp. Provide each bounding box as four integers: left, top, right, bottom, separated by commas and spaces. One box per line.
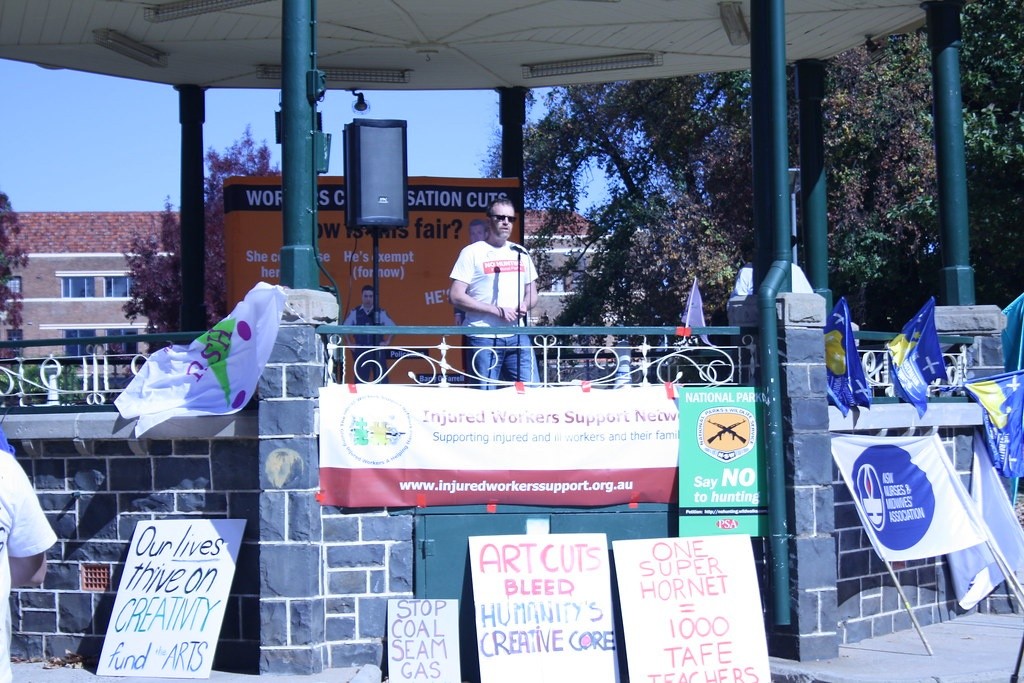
509, 244, 528, 255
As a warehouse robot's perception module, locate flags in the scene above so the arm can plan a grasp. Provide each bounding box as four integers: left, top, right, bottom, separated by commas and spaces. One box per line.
113, 282, 288, 439
942, 425, 1024, 612
824, 295, 871, 418
884, 296, 948, 419
679, 275, 714, 345
939, 292, 1024, 506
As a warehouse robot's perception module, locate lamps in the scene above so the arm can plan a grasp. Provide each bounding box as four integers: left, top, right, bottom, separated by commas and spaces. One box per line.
351, 89, 367, 111
864, 35, 878, 53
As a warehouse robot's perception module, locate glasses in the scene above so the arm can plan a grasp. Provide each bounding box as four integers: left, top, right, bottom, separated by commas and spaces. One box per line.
488, 215, 516, 222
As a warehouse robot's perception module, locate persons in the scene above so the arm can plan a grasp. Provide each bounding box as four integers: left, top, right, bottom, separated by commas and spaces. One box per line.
0, 450, 58, 681
449, 219, 490, 368
448, 199, 540, 390
342, 285, 398, 384
726, 235, 815, 326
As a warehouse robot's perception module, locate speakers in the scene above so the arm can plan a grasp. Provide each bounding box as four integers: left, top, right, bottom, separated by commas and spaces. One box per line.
342, 118, 409, 231
